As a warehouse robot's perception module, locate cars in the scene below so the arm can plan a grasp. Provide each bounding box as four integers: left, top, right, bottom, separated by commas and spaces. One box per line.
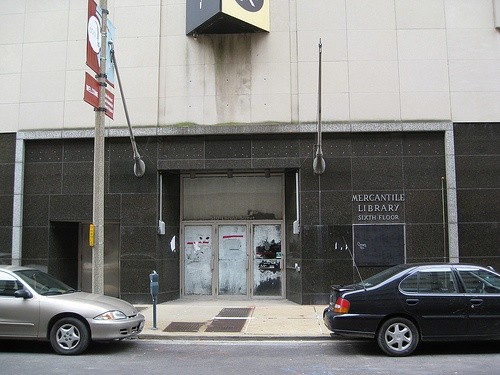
0, 264, 145, 356
322, 261, 500, 355
